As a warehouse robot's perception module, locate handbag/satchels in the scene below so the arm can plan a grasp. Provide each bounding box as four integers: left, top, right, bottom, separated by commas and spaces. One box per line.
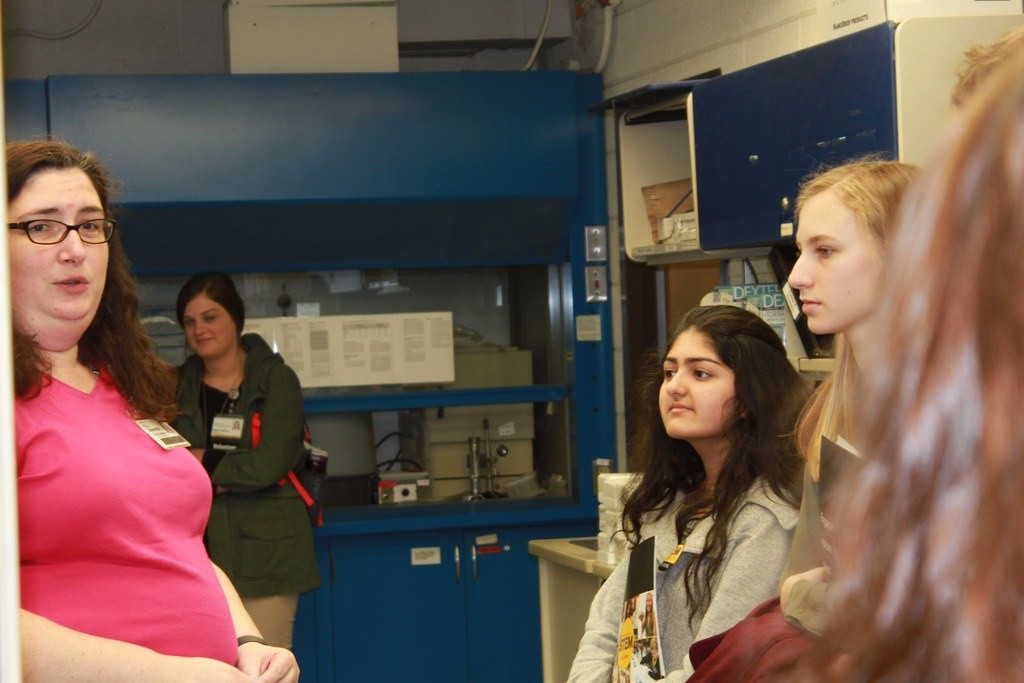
252, 355, 327, 527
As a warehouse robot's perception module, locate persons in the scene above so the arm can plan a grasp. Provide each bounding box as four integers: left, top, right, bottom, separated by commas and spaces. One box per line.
165, 272, 322, 683
2, 136, 300, 683
567, 29, 1024, 683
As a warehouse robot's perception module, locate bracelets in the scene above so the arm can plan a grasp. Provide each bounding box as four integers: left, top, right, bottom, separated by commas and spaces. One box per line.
237, 635, 268, 648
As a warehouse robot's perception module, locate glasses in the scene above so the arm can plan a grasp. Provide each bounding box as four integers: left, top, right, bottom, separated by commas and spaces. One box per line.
8, 218, 117, 245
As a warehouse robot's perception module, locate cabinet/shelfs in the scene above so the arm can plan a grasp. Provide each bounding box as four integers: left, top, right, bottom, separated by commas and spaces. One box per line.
0, 0, 1024, 683
